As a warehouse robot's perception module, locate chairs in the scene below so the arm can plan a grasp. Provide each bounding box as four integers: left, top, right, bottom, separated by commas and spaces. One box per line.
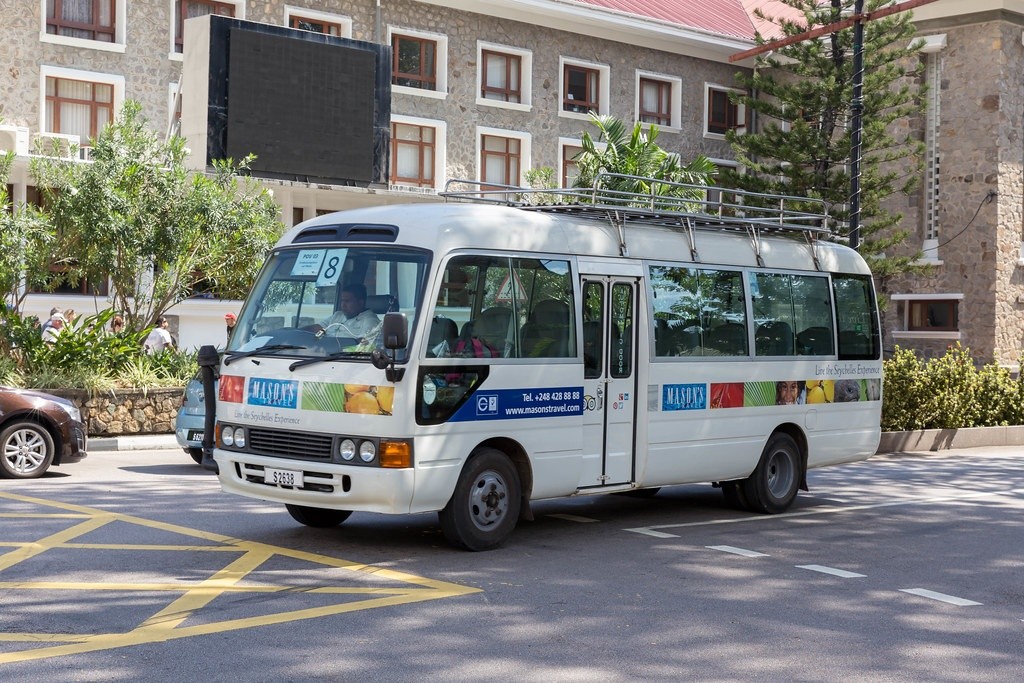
425, 299, 867, 356
365, 295, 399, 314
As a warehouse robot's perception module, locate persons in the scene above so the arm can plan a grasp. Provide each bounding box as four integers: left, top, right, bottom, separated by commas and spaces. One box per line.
222, 312, 238, 347
300, 284, 383, 339
774, 381, 806, 405
1, 301, 180, 359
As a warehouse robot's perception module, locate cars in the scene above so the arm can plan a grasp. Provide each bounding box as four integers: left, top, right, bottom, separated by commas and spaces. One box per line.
175, 346, 227, 463
0, 385, 89, 478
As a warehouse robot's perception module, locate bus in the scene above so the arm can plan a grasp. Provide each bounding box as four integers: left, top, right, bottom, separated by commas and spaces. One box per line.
197, 171, 885, 552
